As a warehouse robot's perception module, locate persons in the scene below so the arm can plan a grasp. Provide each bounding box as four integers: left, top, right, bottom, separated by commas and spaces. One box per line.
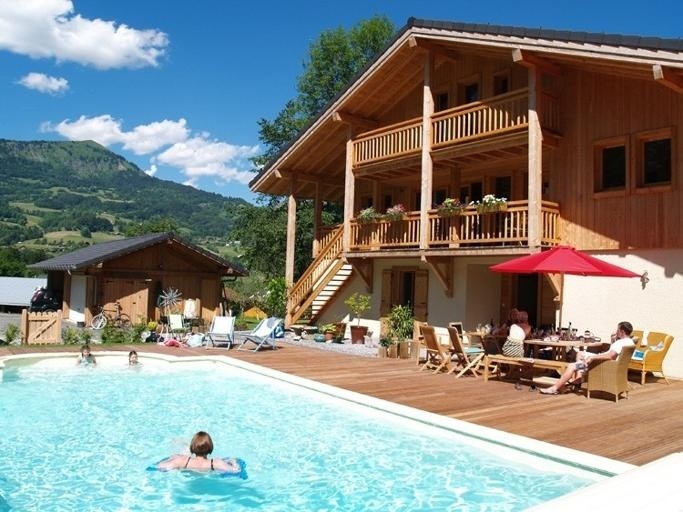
507, 308, 519, 323
502, 311, 531, 377
540, 322, 635, 394
76, 345, 95, 366
160, 432, 241, 475
129, 351, 138, 365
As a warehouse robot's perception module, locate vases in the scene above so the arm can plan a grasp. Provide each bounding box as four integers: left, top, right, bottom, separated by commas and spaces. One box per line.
477, 203, 507, 213
438, 207, 460, 217
391, 215, 402, 221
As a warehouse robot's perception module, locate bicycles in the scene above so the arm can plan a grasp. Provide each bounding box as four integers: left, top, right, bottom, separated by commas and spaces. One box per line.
91, 300, 132, 330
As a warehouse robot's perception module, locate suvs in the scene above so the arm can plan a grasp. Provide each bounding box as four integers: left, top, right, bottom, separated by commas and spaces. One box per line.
30, 288, 61, 314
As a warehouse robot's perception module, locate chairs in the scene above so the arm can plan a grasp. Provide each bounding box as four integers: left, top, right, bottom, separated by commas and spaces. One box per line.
416, 321, 674, 403
168, 314, 187, 341
238, 317, 283, 353
206, 316, 236, 350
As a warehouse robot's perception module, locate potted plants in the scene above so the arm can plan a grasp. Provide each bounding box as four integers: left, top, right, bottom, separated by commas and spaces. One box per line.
389, 304, 416, 358
333, 322, 344, 343
344, 293, 371, 344
320, 323, 336, 340
378, 337, 388, 358
385, 313, 400, 357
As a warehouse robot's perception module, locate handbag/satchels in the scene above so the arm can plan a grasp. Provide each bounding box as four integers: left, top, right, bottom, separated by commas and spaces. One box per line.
502, 340, 524, 357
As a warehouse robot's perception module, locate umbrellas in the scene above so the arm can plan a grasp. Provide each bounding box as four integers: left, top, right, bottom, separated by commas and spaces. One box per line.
489, 246, 642, 330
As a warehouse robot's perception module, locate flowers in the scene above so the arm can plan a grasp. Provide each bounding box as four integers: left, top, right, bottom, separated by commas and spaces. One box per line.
440, 198, 463, 208
386, 204, 406, 217
469, 194, 507, 213
357, 207, 377, 220
